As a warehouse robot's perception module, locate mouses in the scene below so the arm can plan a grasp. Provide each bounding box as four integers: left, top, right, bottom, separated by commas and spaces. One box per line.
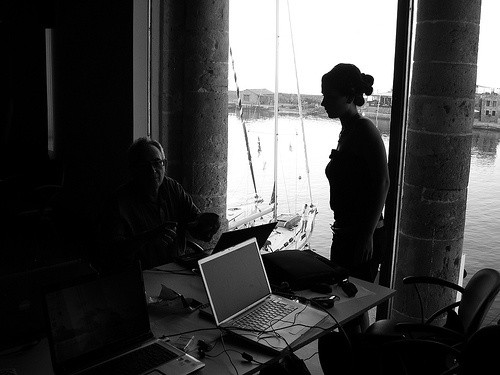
310, 297, 334, 309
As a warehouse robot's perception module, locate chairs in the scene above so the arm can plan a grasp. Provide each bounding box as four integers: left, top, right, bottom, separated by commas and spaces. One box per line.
361, 269, 500, 375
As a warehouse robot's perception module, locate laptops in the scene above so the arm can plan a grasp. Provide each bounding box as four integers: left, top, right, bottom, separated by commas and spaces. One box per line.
173, 220, 278, 272
41, 261, 206, 375
197, 238, 328, 354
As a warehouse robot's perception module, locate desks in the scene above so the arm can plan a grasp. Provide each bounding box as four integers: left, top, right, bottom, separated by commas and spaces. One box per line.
0, 260, 397, 375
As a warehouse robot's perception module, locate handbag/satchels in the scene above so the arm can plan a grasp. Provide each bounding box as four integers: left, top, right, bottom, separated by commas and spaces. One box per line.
262, 249, 350, 294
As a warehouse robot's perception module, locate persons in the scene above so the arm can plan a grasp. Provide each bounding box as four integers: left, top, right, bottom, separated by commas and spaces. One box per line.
101, 137, 219, 272
320, 63, 390, 334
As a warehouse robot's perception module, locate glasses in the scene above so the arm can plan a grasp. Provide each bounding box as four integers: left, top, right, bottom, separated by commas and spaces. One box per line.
155, 159, 165, 167
